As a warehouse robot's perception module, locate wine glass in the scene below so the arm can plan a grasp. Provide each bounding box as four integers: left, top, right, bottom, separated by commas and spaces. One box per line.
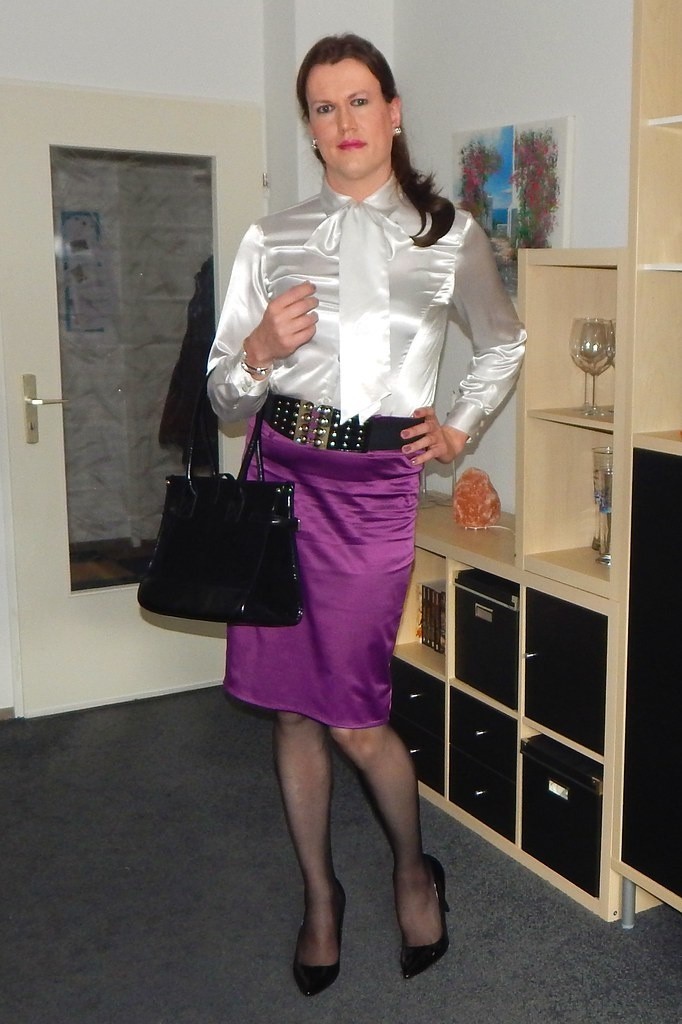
568, 317, 615, 417
576, 323, 612, 413
419, 459, 460, 507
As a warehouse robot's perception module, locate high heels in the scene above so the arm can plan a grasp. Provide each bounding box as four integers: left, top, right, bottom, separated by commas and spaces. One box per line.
292, 878, 347, 996
392, 854, 450, 980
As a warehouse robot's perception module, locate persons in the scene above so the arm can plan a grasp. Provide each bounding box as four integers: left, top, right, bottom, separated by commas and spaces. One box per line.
205, 31, 525, 997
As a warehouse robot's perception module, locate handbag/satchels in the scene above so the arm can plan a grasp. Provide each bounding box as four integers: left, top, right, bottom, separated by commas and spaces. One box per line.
137, 363, 304, 628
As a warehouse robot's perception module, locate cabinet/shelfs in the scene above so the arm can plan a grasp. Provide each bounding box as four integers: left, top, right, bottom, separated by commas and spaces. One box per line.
388, 0, 682, 930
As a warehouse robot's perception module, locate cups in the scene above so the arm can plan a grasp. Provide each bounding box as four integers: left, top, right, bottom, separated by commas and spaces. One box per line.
591, 447, 613, 565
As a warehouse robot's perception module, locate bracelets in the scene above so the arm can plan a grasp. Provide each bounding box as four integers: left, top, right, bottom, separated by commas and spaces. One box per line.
238, 337, 274, 375
